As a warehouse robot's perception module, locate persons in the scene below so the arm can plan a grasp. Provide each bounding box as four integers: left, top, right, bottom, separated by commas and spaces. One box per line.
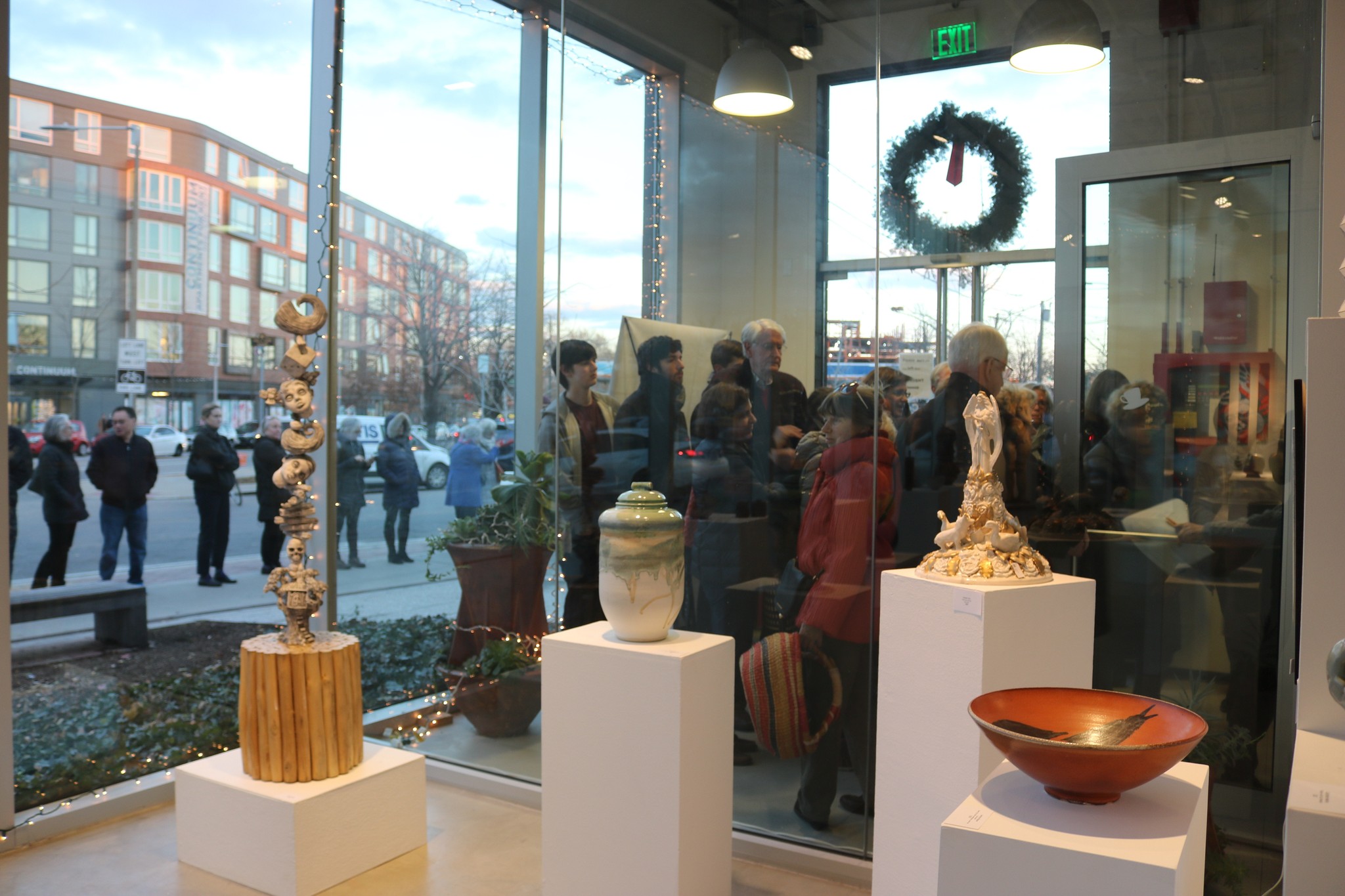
337, 417, 375, 570
8, 426, 34, 583
86, 407, 158, 586
534, 319, 1305, 834
277, 380, 315, 419
270, 455, 316, 491
376, 413, 422, 563
252, 416, 292, 576
445, 415, 515, 525
186, 404, 240, 586
263, 539, 327, 647
31, 413, 89, 589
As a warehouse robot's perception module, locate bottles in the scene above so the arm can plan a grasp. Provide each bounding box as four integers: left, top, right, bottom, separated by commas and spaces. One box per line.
596, 482, 686, 643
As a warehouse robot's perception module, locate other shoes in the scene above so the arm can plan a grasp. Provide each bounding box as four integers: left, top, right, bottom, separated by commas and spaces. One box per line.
399, 552, 413, 562
733, 734, 757, 765
275, 562, 281, 567
794, 802, 827, 830
199, 580, 221, 586
349, 557, 365, 567
337, 554, 349, 568
389, 553, 403, 563
263, 566, 272, 574
735, 710, 754, 731
215, 574, 236, 583
840, 794, 874, 816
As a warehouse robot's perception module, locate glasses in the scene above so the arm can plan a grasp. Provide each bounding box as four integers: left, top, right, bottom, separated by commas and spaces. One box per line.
985, 356, 1013, 378
896, 390, 911, 397
834, 381, 868, 410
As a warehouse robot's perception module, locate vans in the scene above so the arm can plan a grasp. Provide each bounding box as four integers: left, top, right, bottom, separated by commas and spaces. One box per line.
238, 419, 310, 447
336, 414, 451, 490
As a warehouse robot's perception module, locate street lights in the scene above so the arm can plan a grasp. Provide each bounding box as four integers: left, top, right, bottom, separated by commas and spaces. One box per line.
40, 121, 140, 408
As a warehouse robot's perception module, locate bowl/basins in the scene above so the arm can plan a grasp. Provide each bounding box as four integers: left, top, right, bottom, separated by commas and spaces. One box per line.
967, 687, 1209, 806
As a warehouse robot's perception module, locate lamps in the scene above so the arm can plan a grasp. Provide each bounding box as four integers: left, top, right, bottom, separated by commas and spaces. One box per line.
1220, 167, 1235, 183
1183, 53, 1206, 84
711, 37, 794, 117
789, 22, 823, 60
1010, 0, 1104, 74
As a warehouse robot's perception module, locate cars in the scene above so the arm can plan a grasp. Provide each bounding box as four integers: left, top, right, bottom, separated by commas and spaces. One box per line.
132, 426, 188, 457
492, 424, 518, 469
412, 422, 466, 443
90, 427, 118, 447
180, 425, 240, 450
16, 420, 93, 457
540, 430, 733, 495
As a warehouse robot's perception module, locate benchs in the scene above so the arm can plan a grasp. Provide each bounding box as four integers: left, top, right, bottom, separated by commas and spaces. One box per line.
10, 581, 147, 648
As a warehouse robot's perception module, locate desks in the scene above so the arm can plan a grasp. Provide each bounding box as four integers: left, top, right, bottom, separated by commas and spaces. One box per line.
542, 622, 736, 896
871, 567, 1097, 896
937, 758, 1209, 896
174, 741, 429, 896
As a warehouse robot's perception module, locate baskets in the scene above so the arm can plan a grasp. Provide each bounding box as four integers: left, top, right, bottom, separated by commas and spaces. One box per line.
739, 631, 843, 759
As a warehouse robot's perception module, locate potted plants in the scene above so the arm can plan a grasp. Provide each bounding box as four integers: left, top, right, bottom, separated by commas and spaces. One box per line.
425, 449, 573, 739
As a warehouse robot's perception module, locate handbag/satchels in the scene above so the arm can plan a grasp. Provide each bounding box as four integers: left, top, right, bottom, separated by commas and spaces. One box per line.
27, 468, 44, 496
496, 463, 504, 480
187, 454, 213, 478
770, 560, 817, 626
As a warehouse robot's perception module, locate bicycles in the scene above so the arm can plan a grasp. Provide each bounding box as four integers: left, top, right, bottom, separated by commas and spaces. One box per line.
229, 474, 244, 504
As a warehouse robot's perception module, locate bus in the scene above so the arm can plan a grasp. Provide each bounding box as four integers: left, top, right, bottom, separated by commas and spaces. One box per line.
826, 362, 900, 387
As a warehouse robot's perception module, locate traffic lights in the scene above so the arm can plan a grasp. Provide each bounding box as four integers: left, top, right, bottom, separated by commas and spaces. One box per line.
250, 334, 273, 348
